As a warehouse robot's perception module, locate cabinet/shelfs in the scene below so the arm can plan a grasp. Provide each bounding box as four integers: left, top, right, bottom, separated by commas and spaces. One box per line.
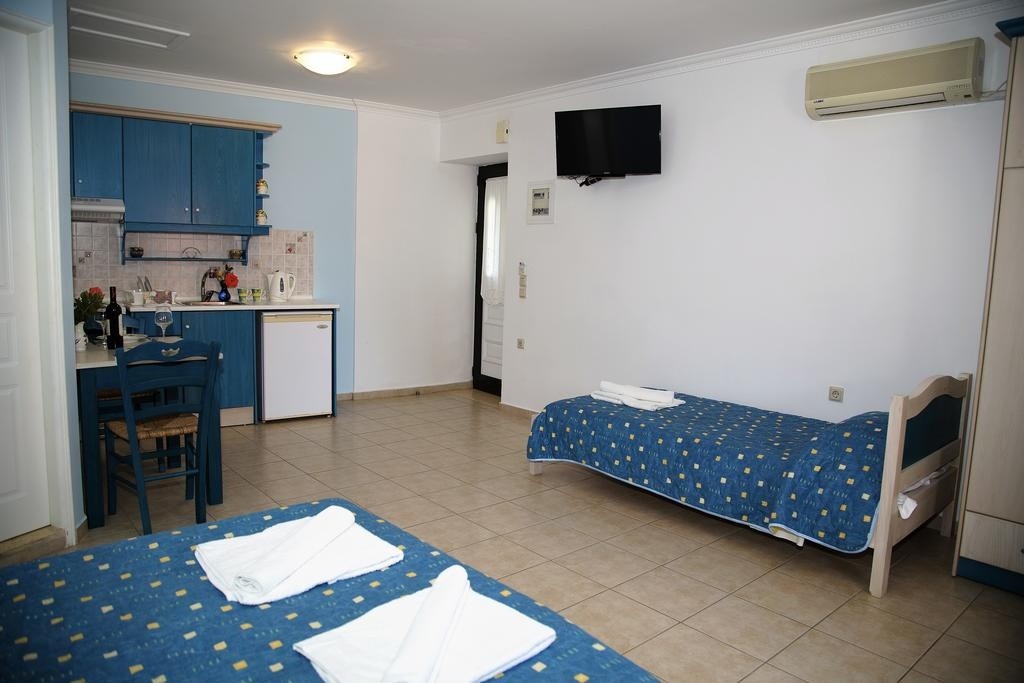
945, 16, 1024, 583
72, 115, 274, 266
74, 311, 257, 430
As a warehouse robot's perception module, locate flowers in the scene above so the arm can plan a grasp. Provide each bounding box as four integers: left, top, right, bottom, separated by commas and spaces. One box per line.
73, 282, 107, 316
209, 264, 241, 289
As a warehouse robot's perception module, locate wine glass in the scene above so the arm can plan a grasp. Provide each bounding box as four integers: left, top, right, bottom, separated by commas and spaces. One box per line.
94, 311, 108, 345
154, 306, 173, 343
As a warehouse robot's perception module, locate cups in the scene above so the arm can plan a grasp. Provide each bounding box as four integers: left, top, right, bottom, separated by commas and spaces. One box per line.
252, 289, 266, 298
229, 249, 243, 259
238, 288, 252, 298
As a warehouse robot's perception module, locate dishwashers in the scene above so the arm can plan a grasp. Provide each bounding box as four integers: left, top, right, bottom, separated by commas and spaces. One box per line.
255, 310, 333, 422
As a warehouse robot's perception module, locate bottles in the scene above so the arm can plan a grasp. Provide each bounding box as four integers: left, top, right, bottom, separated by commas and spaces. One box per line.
105, 287, 122, 350
256, 209, 267, 225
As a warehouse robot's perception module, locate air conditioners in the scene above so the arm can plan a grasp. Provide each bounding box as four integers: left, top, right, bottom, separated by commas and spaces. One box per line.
799, 33, 988, 125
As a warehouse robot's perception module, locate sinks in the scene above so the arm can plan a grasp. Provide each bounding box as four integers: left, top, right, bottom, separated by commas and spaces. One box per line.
176, 299, 245, 307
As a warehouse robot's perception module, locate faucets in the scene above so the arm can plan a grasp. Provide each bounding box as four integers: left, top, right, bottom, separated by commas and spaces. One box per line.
200, 269, 231, 303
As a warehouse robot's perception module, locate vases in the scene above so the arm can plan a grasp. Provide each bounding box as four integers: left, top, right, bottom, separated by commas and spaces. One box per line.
219, 286, 230, 303
82, 319, 104, 344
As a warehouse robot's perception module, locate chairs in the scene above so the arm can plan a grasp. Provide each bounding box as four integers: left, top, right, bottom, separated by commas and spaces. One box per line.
103, 337, 223, 537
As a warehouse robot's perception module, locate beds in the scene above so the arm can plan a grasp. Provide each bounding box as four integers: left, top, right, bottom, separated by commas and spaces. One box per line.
523, 369, 974, 600
0, 494, 666, 683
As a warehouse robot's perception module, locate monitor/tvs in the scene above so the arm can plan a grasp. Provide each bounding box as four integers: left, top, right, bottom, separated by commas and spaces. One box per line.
555, 105, 661, 177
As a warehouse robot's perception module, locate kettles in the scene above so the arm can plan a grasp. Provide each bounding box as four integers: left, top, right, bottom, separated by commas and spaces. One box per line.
267, 269, 296, 302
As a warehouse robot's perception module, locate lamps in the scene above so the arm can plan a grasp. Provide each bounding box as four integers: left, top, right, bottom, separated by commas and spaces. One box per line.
288, 41, 361, 79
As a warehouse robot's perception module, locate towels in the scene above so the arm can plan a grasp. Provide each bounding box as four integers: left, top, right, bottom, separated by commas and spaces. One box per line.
235, 505, 356, 594
591, 391, 686, 411
599, 381, 674, 404
387, 565, 469, 683
290, 563, 557, 683
194, 505, 404, 606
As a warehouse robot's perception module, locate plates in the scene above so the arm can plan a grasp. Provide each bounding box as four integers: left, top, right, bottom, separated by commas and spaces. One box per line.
123, 334, 147, 344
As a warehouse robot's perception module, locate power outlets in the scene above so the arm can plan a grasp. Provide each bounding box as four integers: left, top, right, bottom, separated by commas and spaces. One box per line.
514, 336, 527, 350
827, 383, 846, 403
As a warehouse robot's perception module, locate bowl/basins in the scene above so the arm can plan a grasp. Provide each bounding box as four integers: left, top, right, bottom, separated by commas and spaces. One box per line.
131, 247, 143, 257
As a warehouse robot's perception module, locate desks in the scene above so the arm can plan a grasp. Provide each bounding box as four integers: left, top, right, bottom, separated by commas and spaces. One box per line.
74, 332, 224, 531
